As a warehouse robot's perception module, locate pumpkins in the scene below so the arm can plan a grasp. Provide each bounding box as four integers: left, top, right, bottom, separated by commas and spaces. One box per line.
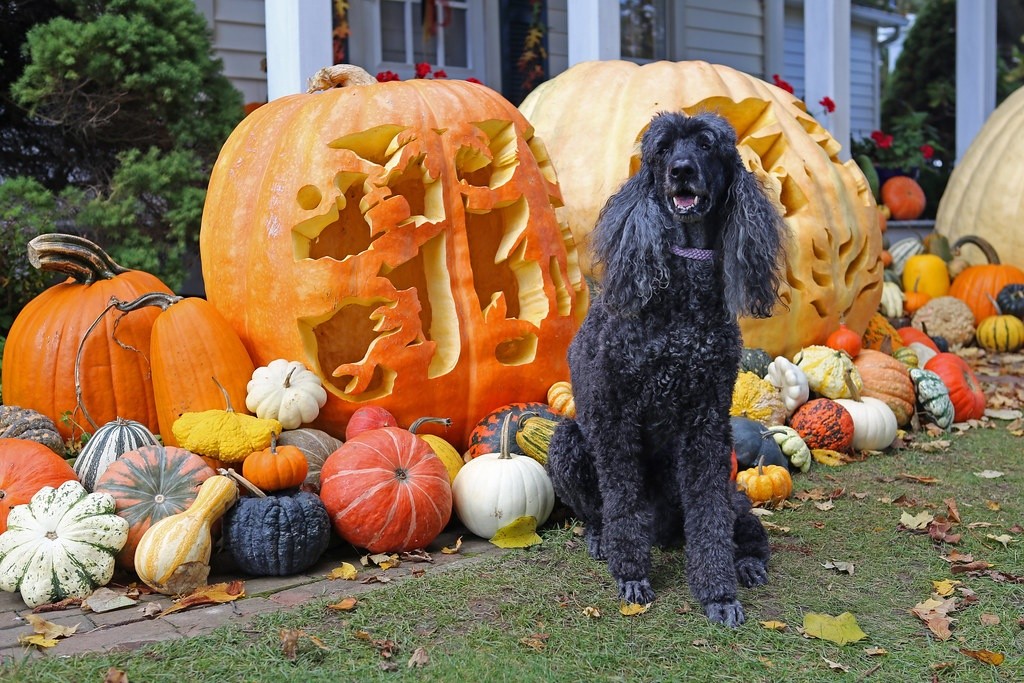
0, 85, 1024, 609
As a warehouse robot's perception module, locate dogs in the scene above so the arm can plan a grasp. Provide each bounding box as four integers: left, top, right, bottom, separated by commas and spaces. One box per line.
546, 107, 799, 626
